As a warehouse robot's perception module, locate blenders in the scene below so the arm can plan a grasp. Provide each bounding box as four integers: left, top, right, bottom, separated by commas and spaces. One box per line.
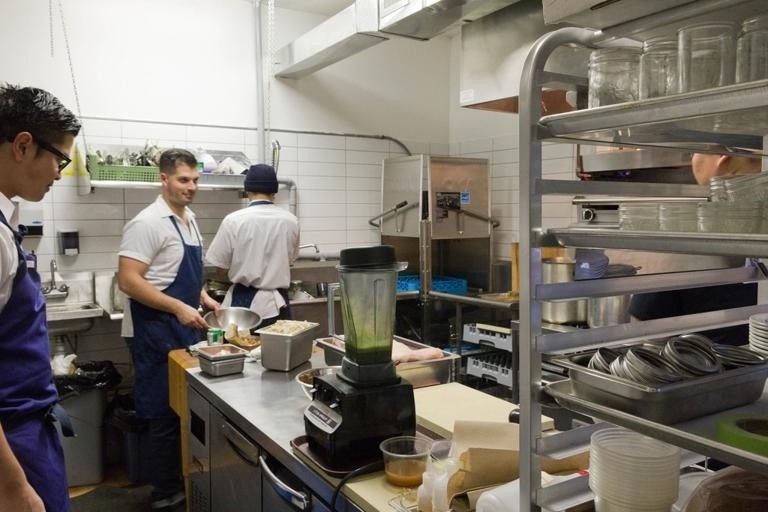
303, 245, 419, 479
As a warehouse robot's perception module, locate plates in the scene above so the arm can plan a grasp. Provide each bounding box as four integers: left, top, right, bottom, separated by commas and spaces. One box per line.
747, 312, 768, 358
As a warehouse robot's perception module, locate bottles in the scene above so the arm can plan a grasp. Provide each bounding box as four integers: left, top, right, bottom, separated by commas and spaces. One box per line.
638, 35, 679, 96
736, 15, 767, 85
475, 465, 588, 512
586, 47, 643, 109
417, 456, 466, 512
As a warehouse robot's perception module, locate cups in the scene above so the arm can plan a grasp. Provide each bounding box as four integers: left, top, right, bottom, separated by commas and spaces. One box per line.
677, 18, 736, 96
316, 281, 329, 298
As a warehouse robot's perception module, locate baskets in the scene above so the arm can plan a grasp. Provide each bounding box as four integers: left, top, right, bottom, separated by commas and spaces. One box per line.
86, 155, 160, 182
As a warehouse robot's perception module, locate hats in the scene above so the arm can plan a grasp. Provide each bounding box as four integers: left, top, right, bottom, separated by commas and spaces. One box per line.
244, 165, 277, 194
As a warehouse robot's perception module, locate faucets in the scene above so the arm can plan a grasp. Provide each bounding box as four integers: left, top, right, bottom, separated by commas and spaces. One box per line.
50, 259, 58, 289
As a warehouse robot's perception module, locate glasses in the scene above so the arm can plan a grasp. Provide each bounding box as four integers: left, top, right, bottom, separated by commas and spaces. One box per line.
10, 135, 70, 174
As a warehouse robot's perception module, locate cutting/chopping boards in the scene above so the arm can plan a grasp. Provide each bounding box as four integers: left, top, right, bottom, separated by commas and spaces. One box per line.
412, 382, 555, 438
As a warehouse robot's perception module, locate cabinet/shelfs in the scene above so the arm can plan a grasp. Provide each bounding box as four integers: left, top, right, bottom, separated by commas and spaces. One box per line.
517, 26, 768, 512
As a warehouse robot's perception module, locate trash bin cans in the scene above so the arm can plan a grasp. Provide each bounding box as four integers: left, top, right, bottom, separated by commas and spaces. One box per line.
50, 360, 151, 488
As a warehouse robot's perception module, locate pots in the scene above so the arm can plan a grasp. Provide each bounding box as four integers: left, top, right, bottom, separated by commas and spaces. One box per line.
541, 257, 640, 329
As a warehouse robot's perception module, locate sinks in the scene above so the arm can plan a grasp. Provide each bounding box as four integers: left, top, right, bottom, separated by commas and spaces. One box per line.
45, 302, 103, 322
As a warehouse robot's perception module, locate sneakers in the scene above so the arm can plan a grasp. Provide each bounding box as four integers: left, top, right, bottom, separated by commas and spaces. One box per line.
151, 484, 186, 511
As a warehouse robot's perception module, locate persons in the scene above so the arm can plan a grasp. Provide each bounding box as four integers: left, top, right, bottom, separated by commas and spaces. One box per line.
205, 163, 302, 335
0, 83, 81, 512
118, 149, 221, 511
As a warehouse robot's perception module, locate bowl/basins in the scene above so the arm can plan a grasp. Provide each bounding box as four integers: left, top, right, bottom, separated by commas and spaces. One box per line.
295, 364, 344, 402
616, 167, 768, 232
201, 305, 262, 336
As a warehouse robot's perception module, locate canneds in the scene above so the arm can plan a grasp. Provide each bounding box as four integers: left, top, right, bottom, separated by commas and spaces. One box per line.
207, 327, 224, 346
